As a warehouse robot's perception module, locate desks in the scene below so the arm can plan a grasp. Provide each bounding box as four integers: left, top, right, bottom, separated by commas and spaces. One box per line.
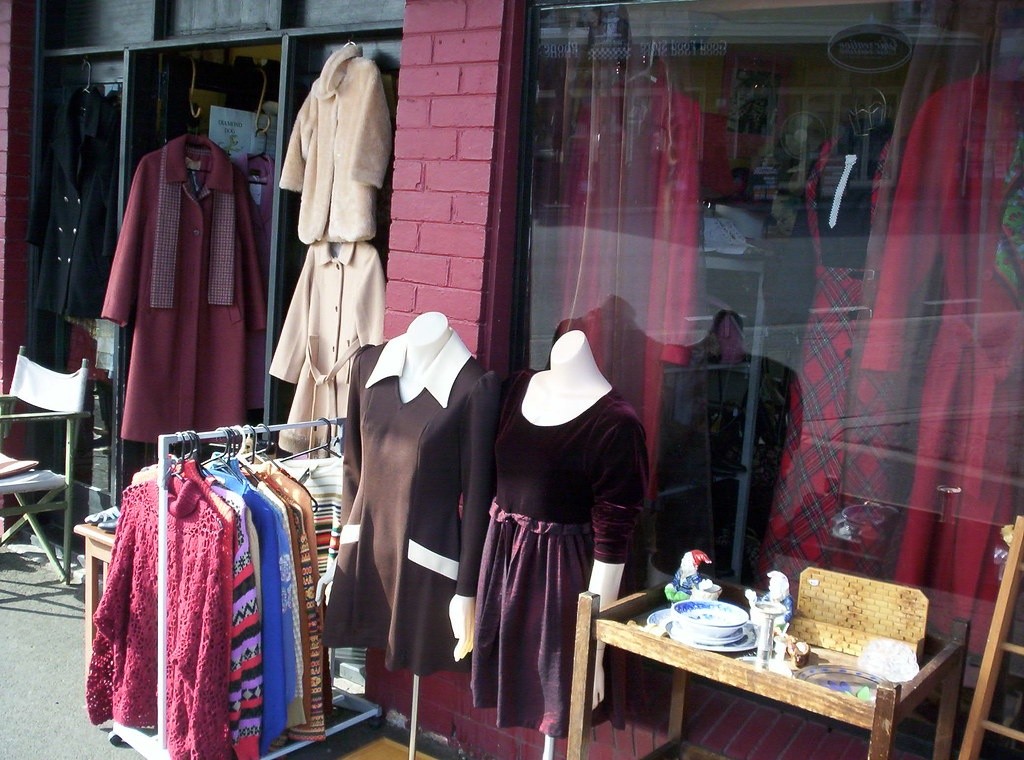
73, 523, 115, 679
566, 577, 971, 760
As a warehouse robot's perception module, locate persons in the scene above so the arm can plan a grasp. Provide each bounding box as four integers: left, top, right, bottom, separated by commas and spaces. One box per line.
467, 328, 649, 738
315, 312, 498, 676
549, 295, 801, 591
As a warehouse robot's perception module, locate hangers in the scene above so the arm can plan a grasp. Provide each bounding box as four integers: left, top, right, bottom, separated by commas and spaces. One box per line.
80, 60, 92, 110
170, 417, 342, 513
248, 128, 268, 185
627, 38, 657, 124
185, 114, 209, 173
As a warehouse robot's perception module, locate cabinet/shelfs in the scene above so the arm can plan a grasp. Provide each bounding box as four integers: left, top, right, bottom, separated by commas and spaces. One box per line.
660, 257, 768, 586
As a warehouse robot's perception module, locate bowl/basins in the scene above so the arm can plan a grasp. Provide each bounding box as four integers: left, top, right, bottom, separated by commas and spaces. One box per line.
670, 600, 749, 637
841, 505, 899, 531
737, 657, 793, 680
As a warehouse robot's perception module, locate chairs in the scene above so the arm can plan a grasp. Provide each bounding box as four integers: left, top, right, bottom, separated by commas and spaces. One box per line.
0, 346, 91, 587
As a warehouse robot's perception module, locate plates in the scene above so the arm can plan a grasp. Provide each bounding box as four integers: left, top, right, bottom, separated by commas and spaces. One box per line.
647, 609, 757, 652
794, 665, 888, 704
833, 520, 861, 546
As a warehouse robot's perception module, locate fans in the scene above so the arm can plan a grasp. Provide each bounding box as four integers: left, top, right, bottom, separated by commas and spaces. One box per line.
778, 110, 828, 174
